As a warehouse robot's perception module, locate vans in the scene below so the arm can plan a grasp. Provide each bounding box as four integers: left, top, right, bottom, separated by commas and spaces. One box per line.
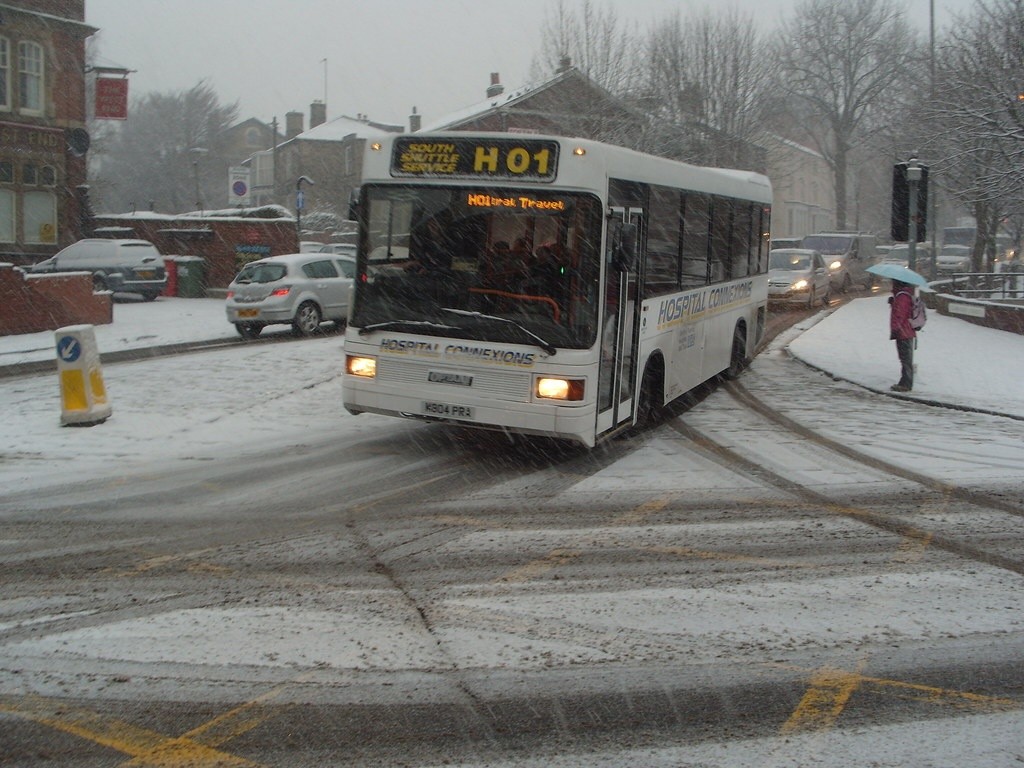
799, 233, 877, 293
768, 238, 803, 251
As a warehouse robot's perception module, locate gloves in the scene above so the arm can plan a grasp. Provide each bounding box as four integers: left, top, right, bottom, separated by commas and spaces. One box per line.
891, 332, 897, 340
888, 297, 894, 303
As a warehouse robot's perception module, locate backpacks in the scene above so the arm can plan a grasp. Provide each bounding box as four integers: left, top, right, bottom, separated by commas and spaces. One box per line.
895, 291, 927, 331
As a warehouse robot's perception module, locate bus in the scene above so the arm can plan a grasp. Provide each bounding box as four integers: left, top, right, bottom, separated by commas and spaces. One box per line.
340, 133, 772, 450
943, 228, 980, 250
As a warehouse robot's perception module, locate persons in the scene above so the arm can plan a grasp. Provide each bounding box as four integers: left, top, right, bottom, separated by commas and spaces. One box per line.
888, 279, 916, 391
405, 205, 562, 302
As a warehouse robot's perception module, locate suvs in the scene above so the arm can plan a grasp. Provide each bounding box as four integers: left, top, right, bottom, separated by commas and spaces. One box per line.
881, 243, 936, 280
24, 238, 169, 302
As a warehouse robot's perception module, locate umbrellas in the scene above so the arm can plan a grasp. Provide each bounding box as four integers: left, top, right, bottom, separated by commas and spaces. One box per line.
865, 263, 927, 288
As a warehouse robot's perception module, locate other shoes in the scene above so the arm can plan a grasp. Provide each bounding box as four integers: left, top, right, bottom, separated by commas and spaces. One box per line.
891, 384, 912, 392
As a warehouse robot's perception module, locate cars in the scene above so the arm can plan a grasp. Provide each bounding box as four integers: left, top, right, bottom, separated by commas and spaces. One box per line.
225, 253, 382, 338
299, 241, 411, 274
936, 245, 972, 273
768, 249, 832, 309
